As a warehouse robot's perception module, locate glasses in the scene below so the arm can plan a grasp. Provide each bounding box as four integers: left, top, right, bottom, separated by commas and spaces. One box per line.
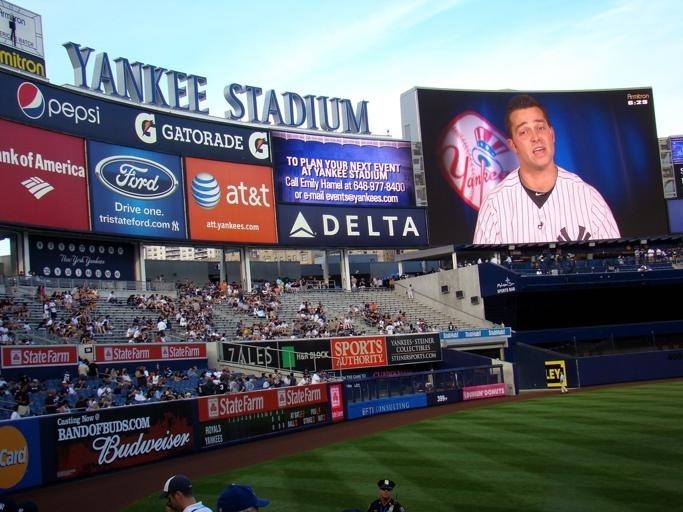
380, 487, 393, 491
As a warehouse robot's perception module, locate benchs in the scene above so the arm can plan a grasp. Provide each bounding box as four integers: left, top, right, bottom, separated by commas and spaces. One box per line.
484, 246, 683, 286
1, 280, 508, 421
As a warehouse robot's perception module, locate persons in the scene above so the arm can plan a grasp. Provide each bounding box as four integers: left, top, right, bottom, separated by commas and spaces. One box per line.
367, 479, 406, 512
473, 94, 621, 244
1, 270, 460, 420
427, 249, 682, 276
160, 475, 213, 512
20, 501, 39, 512
218, 483, 269, 512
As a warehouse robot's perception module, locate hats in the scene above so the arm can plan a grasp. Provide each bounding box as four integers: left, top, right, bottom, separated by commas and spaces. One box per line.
375, 478, 396, 490
16, 499, 38, 512
214, 481, 270, 512
158, 473, 194, 500
